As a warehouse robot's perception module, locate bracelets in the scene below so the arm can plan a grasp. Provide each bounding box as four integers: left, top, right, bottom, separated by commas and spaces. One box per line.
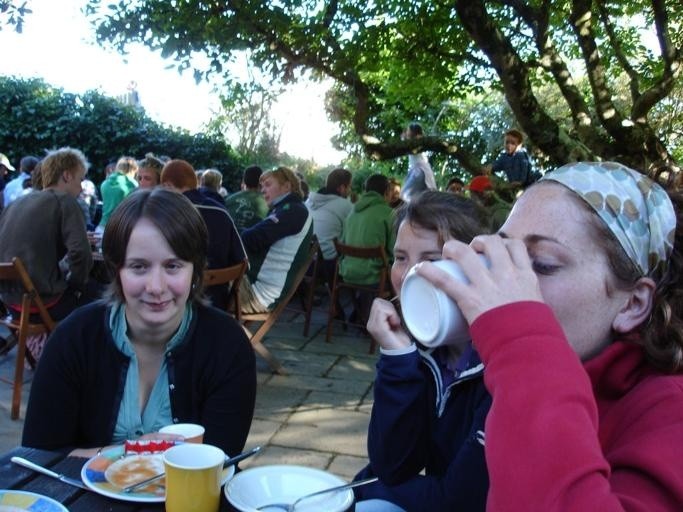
97, 446, 103, 456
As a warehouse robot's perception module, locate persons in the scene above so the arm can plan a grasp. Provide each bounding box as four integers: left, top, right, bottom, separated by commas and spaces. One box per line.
411, 162, 683, 512
21, 188, 257, 457
1, 146, 312, 371
306, 125, 541, 324
352, 190, 497, 512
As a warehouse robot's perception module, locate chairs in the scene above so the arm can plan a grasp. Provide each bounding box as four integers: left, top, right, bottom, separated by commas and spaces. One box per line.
2, 257, 67, 419
192, 257, 245, 325
230, 235, 319, 377
287, 241, 337, 338
326, 238, 394, 354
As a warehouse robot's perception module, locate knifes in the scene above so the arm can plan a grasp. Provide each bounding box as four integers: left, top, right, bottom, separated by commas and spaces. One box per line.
11, 457, 91, 491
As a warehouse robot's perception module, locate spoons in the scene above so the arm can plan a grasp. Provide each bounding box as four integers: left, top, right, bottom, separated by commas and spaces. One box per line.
257, 478, 379, 511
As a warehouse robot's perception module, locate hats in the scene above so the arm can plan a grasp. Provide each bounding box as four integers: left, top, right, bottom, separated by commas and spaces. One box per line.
0, 153, 16, 172
463, 176, 492, 193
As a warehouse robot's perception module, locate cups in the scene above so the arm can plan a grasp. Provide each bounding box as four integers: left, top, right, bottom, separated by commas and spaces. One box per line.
400, 253, 486, 348
159, 423, 205, 444
163, 443, 225, 512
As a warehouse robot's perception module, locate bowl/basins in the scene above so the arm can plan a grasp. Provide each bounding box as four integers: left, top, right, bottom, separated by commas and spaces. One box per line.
225, 465, 354, 511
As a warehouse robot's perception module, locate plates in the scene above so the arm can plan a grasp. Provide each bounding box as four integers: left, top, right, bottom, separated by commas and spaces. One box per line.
0, 490, 69, 511
81, 440, 235, 503
